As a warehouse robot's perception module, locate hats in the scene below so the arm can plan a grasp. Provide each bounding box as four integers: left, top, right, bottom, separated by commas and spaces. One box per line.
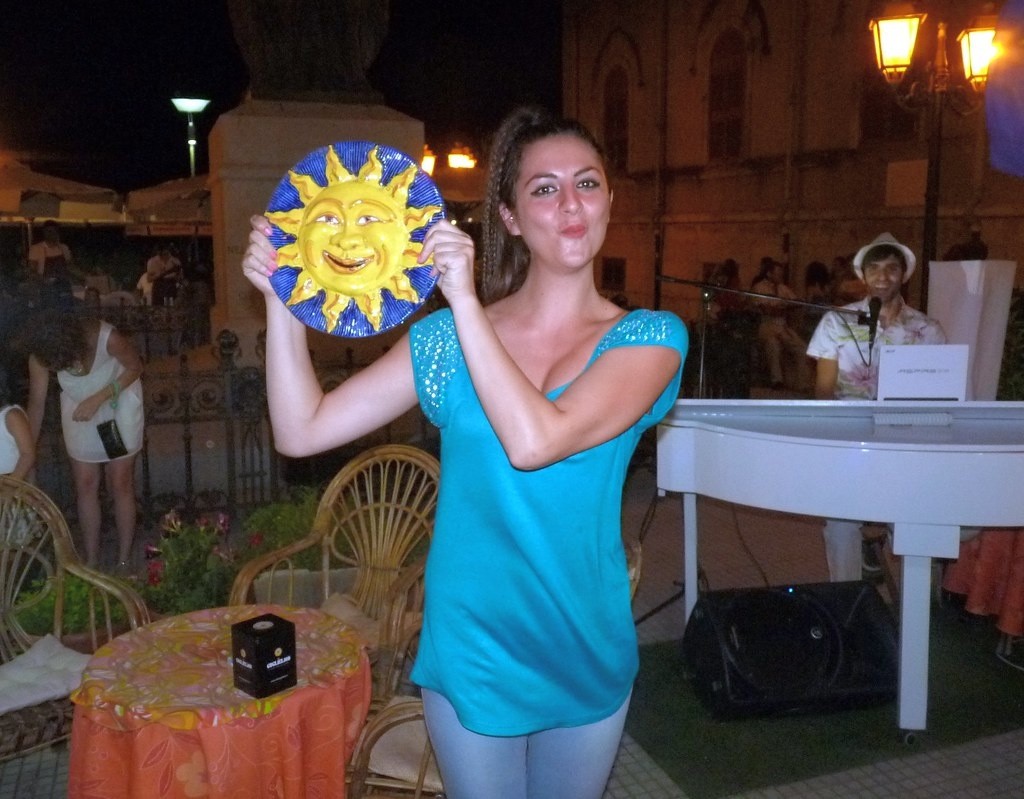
854, 232, 917, 283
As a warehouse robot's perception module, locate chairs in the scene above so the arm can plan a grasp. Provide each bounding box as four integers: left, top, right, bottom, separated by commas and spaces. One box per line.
346, 694, 445, 799
0, 476, 154, 761
227, 443, 440, 725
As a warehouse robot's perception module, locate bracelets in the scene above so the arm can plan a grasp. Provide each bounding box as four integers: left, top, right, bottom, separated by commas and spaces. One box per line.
109, 384, 113, 398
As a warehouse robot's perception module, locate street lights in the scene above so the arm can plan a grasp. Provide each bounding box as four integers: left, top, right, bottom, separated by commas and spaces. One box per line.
868, 2, 1012, 313
171, 97, 211, 175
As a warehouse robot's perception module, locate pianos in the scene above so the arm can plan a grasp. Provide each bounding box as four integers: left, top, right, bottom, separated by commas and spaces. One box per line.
657, 401, 1024, 732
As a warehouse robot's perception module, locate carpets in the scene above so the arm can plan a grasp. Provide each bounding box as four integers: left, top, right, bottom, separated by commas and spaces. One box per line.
623, 582, 1024, 799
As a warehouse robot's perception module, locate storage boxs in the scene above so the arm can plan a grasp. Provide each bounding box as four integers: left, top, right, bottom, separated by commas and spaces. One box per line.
230, 614, 296, 700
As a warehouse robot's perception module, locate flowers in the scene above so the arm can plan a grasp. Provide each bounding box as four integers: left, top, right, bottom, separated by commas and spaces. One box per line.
140, 498, 264, 616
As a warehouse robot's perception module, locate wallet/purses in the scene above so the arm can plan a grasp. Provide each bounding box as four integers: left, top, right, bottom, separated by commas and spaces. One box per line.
97, 420, 128, 459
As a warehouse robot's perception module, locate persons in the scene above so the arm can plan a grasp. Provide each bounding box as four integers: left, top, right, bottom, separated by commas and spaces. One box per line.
0, 346, 41, 575
242, 106, 690, 799
24, 307, 146, 580
807, 231, 983, 582
942, 214, 989, 260
711, 253, 871, 383
14, 220, 101, 334
750, 262, 810, 392
146, 247, 184, 282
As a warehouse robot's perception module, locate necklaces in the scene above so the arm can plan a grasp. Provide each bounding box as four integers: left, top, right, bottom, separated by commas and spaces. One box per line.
67, 360, 84, 375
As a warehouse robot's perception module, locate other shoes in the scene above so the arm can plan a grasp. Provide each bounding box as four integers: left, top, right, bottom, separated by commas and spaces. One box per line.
995, 633, 1024, 671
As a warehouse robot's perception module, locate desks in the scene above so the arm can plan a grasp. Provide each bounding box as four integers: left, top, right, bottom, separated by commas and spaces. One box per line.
66, 602, 372, 799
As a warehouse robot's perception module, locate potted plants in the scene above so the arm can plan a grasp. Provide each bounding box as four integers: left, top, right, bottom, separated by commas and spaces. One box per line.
240, 477, 433, 608
12, 573, 133, 656
